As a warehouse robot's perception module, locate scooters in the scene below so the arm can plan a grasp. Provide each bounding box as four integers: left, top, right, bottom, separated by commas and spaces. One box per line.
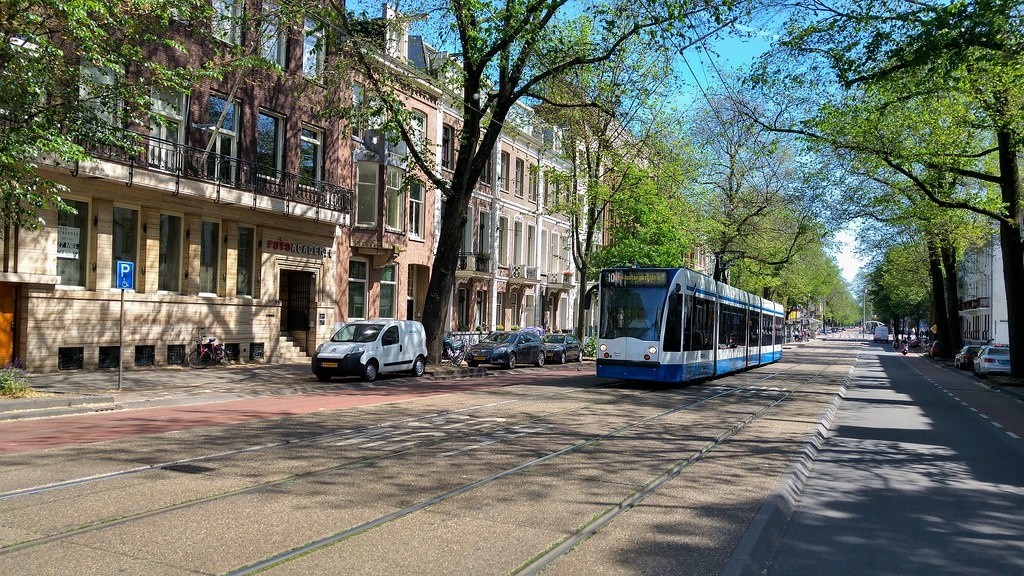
902, 345, 908, 355
894, 342, 898, 350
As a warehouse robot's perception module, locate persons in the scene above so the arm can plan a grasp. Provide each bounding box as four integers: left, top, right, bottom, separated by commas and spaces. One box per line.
628, 308, 651, 328
793, 330, 810, 341
893, 336, 899, 342
902, 337, 908, 344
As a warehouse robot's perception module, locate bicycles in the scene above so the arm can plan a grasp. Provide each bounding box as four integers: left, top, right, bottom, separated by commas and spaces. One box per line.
187, 327, 229, 369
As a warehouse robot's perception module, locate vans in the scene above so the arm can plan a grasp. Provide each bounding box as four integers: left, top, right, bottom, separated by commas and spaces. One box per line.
312, 319, 428, 382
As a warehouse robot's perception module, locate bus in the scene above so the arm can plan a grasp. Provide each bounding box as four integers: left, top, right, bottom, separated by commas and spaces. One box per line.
584, 257, 785, 390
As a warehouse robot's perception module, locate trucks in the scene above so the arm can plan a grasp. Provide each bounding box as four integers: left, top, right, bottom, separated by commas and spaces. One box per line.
873, 326, 889, 343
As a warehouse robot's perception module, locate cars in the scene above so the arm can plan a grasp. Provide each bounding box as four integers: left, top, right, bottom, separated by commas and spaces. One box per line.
541, 333, 583, 364
954, 345, 981, 371
929, 340, 941, 359
463, 330, 547, 369
972, 342, 1012, 379
820, 327, 845, 334
